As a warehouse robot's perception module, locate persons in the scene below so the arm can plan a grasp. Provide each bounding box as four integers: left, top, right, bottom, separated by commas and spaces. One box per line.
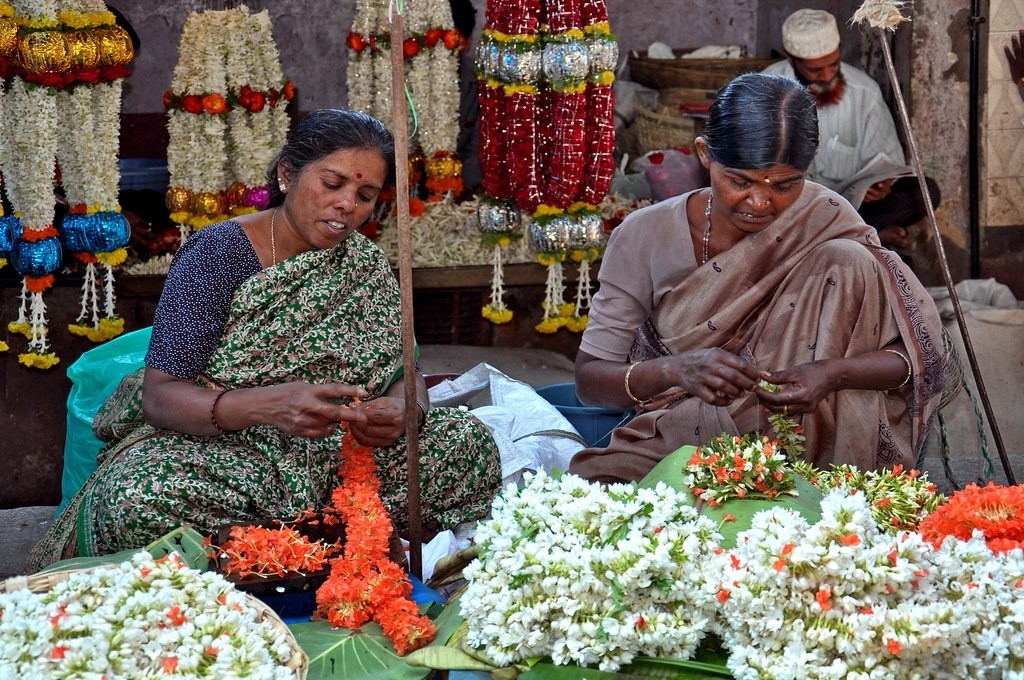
759, 8, 942, 250
565, 72, 965, 484
30, 108, 503, 593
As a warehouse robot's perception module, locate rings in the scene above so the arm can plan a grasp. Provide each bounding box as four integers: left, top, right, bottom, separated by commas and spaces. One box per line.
781, 405, 788, 416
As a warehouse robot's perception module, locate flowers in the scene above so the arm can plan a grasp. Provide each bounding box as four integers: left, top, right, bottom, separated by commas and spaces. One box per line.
1, 382, 1024, 680
0, 1, 617, 370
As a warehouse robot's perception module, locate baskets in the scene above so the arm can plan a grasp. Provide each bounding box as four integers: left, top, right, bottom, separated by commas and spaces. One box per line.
627, 47, 787, 156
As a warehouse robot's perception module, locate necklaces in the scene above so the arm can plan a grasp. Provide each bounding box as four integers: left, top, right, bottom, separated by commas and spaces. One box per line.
269, 209, 282, 265
702, 191, 714, 266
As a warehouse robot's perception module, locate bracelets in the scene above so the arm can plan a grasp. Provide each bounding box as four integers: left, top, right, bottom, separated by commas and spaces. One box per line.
624, 359, 652, 403
209, 386, 235, 434
416, 401, 427, 428
885, 349, 912, 390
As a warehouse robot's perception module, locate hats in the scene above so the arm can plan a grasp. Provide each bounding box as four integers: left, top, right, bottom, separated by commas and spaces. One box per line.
781, 9, 841, 59
